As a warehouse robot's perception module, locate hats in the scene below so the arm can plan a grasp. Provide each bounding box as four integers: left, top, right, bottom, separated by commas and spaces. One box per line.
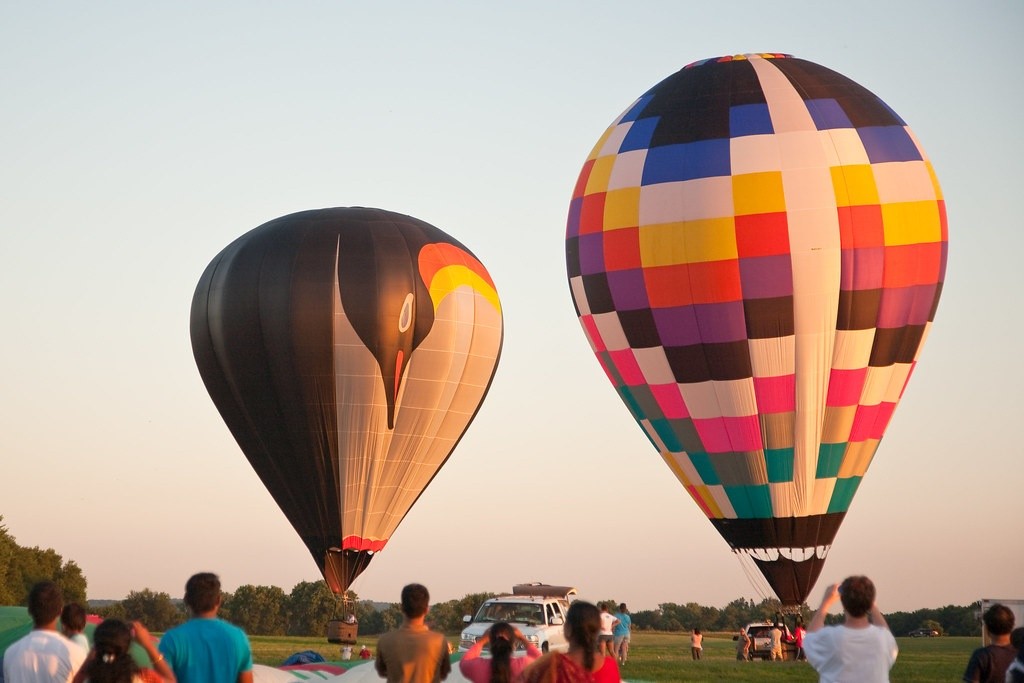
361, 645, 366, 649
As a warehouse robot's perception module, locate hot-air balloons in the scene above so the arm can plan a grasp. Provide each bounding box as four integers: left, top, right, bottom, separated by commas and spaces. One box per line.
188, 206, 505, 647
564, 51, 950, 664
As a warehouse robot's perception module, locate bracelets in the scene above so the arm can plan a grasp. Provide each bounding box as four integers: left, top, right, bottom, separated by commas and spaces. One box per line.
150, 655, 165, 665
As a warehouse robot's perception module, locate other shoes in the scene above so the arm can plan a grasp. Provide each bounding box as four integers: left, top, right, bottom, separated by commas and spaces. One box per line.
619, 657, 621, 660
621, 662, 625, 665
625, 658, 627, 660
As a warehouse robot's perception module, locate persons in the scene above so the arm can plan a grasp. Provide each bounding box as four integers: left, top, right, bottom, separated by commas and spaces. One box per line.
458, 602, 631, 683
376, 583, 451, 682
803, 576, 898, 683
2, 572, 254, 683
737, 628, 751, 662
962, 604, 1024, 683
691, 629, 704, 658
340, 644, 373, 662
769, 617, 804, 660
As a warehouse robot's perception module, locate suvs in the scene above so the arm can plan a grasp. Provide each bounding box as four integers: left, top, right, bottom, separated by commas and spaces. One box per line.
455, 581, 577, 656
731, 620, 795, 660
